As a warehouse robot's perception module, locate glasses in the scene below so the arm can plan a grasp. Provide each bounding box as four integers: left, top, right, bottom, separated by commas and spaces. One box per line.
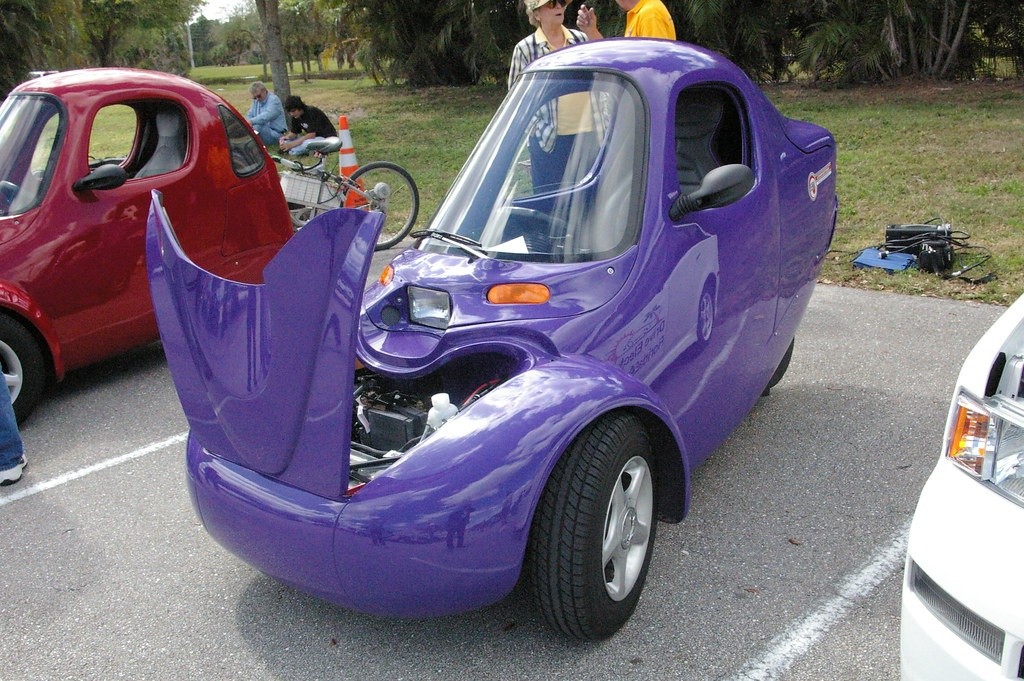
252, 92, 263, 100
533, 0, 566, 12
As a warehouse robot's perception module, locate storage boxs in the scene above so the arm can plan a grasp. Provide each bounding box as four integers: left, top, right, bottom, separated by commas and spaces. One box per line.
281, 171, 342, 209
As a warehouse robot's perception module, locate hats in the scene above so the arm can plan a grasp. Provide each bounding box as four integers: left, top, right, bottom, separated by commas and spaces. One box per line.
532, 0, 572, 11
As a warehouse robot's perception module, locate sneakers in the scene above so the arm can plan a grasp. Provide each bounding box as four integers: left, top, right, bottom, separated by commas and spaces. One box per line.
0, 454, 27, 486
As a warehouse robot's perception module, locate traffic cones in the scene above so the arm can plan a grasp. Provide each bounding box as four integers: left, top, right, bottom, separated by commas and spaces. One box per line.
337, 115, 370, 214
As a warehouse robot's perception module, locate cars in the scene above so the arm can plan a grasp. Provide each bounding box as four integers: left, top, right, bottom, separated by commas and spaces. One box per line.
0, 67, 293, 432
897, 294, 1024, 681
146, 39, 838, 644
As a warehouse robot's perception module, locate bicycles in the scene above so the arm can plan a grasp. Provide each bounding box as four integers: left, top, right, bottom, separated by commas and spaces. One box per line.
269, 137, 421, 252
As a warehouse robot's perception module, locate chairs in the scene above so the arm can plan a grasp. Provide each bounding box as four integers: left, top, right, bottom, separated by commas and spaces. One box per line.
136, 107, 183, 179
676, 91, 727, 194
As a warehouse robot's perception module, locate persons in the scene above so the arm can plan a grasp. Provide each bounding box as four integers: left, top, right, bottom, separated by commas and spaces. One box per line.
508, 0, 611, 197
279, 96, 337, 156
576, 0, 676, 40
246, 82, 288, 145
0, 365, 28, 484
